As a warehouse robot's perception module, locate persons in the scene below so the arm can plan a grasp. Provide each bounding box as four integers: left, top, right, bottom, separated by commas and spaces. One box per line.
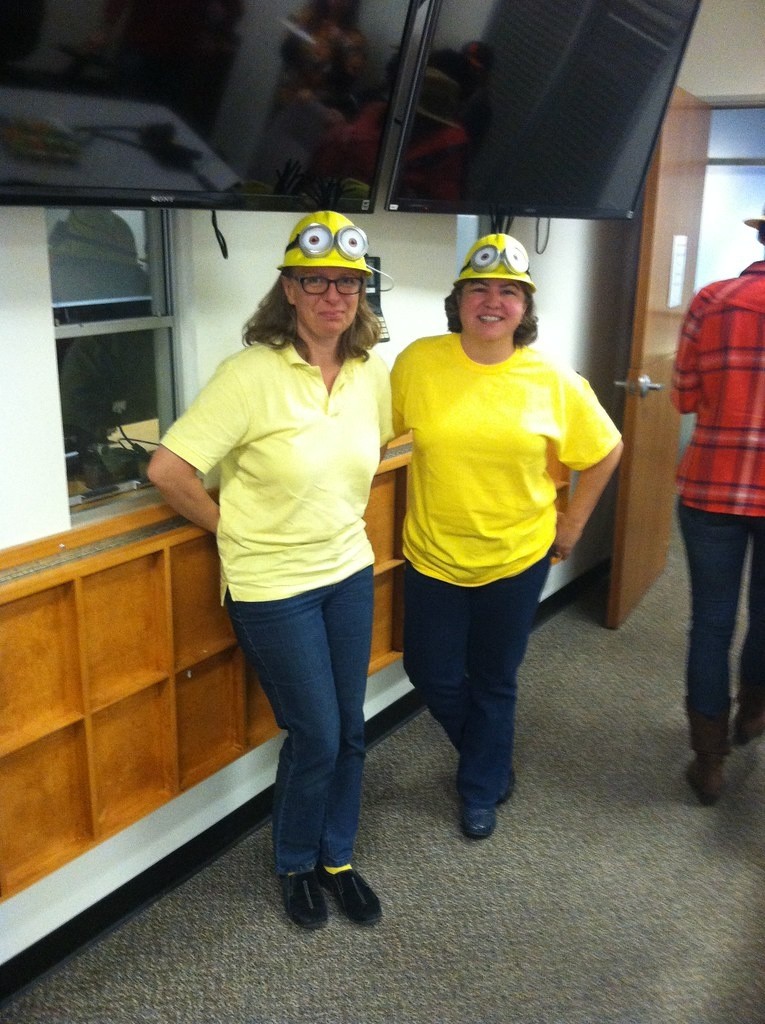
145, 211, 398, 928
49, 209, 155, 440
275, 0, 490, 202
672, 201, 765, 807
391, 233, 623, 838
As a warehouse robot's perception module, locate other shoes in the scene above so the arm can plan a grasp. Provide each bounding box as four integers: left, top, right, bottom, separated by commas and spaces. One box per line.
280, 868, 325, 929
498, 769, 516, 804
457, 802, 498, 837
320, 864, 382, 924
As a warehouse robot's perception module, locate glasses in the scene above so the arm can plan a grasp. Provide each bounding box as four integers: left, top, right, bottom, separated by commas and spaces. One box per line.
299, 222, 369, 261
470, 244, 529, 276
290, 273, 364, 295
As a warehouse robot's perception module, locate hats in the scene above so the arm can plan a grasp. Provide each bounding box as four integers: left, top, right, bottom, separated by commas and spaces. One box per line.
743, 216, 765, 228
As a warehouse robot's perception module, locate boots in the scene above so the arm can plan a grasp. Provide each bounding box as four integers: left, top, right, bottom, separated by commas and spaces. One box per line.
681, 696, 726, 803
733, 679, 764, 743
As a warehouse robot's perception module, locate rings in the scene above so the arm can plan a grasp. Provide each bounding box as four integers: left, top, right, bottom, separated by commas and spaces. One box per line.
553, 552, 561, 558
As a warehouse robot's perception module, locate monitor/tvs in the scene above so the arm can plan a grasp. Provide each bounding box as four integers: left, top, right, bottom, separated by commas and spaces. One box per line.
48, 254, 160, 493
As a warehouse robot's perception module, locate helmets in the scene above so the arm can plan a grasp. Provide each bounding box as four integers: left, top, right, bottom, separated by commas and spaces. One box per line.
279, 211, 373, 276
452, 233, 537, 293
